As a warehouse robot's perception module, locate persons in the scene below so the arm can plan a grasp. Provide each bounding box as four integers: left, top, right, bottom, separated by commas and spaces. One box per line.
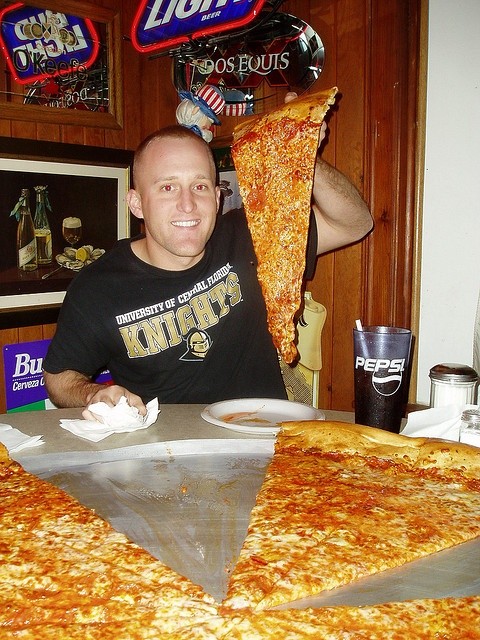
42, 92, 374, 421
220, 89, 254, 117
175, 84, 226, 143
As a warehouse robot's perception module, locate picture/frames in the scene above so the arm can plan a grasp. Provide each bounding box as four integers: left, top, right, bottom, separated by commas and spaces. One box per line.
1, 1, 125, 132
1, 135, 140, 331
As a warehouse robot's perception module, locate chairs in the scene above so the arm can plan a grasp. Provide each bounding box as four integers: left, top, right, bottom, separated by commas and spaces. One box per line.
266, 293, 327, 408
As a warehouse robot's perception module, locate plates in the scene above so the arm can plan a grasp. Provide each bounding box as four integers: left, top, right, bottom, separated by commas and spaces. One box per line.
56, 253, 81, 271
199, 397, 324, 437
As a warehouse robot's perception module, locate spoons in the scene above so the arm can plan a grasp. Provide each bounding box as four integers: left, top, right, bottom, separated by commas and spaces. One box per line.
41, 261, 69, 280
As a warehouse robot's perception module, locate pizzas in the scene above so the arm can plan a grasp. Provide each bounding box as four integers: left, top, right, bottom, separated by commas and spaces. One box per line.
1, 421, 480, 639
228, 87, 340, 366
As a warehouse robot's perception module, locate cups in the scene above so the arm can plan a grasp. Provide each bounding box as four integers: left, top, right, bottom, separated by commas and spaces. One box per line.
354, 325, 411, 435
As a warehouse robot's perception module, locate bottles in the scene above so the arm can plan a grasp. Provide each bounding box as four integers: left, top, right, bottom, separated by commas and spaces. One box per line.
429, 362, 478, 408
459, 410, 480, 447
34, 188, 53, 265
16, 189, 38, 271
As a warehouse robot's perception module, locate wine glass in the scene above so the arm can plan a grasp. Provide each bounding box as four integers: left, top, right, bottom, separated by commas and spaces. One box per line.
62, 216, 83, 247
